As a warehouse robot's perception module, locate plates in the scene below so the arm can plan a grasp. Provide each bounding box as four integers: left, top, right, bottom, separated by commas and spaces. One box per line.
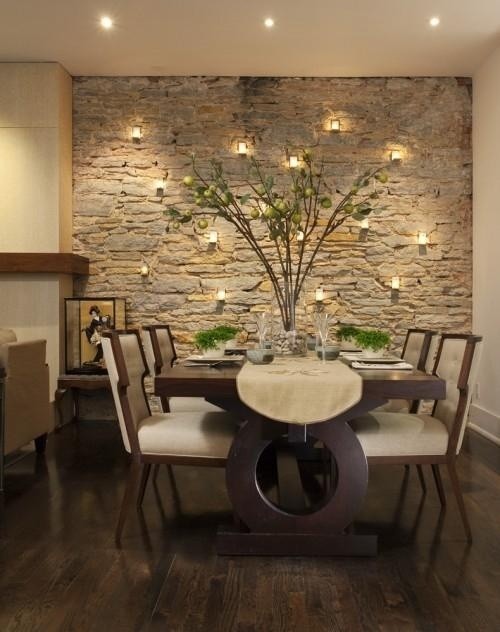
185, 355, 244, 365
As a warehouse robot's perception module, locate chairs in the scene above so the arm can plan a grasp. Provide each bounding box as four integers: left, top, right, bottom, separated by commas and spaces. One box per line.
141, 325, 227, 413
314, 333, 483, 545
370, 327, 439, 414
100, 329, 269, 551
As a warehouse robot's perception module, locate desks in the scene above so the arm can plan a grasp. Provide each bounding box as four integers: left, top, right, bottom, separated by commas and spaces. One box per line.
54, 374, 112, 429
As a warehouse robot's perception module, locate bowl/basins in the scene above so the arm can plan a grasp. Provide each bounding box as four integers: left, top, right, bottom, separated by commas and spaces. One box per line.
246, 349, 276, 364
318, 346, 339, 361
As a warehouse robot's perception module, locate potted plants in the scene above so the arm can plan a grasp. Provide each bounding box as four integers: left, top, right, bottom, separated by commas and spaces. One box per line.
194, 330, 224, 357
163, 144, 401, 358
213, 326, 239, 348
355, 330, 390, 357
336, 326, 360, 348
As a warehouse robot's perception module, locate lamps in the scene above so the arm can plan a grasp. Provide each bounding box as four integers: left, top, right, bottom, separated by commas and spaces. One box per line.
418, 233, 427, 244
209, 230, 217, 244
290, 153, 298, 168
331, 119, 340, 133
392, 276, 400, 290
297, 228, 304, 242
140, 263, 148, 276
238, 140, 248, 156
217, 287, 225, 301
156, 179, 164, 190
361, 216, 370, 229
131, 124, 142, 140
316, 288, 323, 301
392, 149, 401, 161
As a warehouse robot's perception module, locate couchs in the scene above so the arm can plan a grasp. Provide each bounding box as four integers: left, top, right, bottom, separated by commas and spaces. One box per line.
0, 328, 50, 493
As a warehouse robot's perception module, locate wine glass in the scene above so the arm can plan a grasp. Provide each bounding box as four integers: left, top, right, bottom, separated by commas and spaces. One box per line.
254, 313, 272, 350
314, 313, 333, 365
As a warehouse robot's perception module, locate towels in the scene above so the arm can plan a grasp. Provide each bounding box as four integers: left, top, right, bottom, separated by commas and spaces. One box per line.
339, 352, 362, 356
351, 362, 414, 370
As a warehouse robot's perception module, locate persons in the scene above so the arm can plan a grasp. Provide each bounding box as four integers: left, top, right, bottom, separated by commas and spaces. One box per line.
81, 305, 115, 362
